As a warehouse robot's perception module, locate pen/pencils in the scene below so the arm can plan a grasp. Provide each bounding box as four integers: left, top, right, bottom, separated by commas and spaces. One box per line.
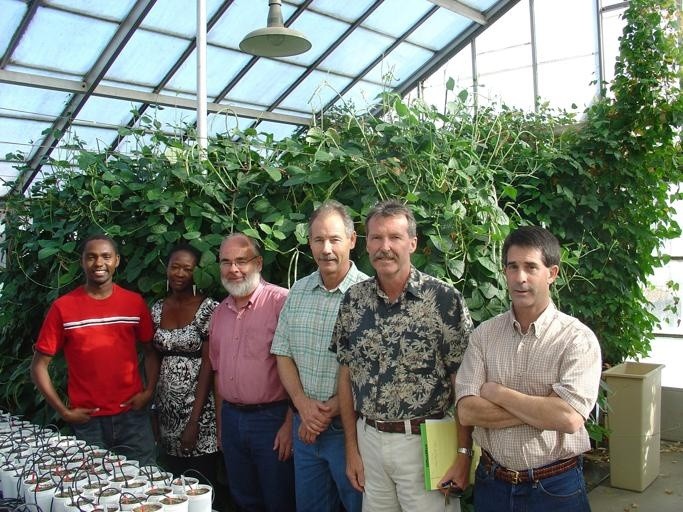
441, 479, 453, 487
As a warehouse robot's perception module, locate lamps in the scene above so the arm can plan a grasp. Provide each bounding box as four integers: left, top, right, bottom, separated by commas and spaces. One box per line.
239, 1, 311, 57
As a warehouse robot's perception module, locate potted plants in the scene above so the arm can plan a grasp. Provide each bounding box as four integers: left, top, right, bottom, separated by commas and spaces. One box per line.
0, 405, 212, 511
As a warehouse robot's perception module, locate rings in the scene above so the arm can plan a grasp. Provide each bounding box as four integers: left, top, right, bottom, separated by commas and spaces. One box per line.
290, 448, 294, 452
184, 448, 189, 451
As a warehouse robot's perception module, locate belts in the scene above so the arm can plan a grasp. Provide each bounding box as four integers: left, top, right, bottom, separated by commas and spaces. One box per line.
480, 449, 578, 485
228, 400, 289, 411
287, 398, 298, 414
359, 412, 446, 434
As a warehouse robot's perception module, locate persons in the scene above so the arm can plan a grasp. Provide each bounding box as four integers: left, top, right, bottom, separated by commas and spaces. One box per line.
149, 244, 221, 486
270, 200, 373, 512
31, 236, 161, 468
328, 200, 475, 512
208, 233, 292, 511
454, 225, 603, 512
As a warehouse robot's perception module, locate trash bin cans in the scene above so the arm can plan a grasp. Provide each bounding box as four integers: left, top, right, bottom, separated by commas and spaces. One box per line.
602, 361, 666, 493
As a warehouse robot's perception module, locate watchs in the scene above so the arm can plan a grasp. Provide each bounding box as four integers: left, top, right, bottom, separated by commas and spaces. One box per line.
455, 447, 476, 458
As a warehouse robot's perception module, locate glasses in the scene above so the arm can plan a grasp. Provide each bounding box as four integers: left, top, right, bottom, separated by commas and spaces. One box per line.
222, 256, 258, 266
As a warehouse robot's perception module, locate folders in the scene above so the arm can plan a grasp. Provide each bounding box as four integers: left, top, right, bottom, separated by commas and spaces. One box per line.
419, 418, 481, 491
